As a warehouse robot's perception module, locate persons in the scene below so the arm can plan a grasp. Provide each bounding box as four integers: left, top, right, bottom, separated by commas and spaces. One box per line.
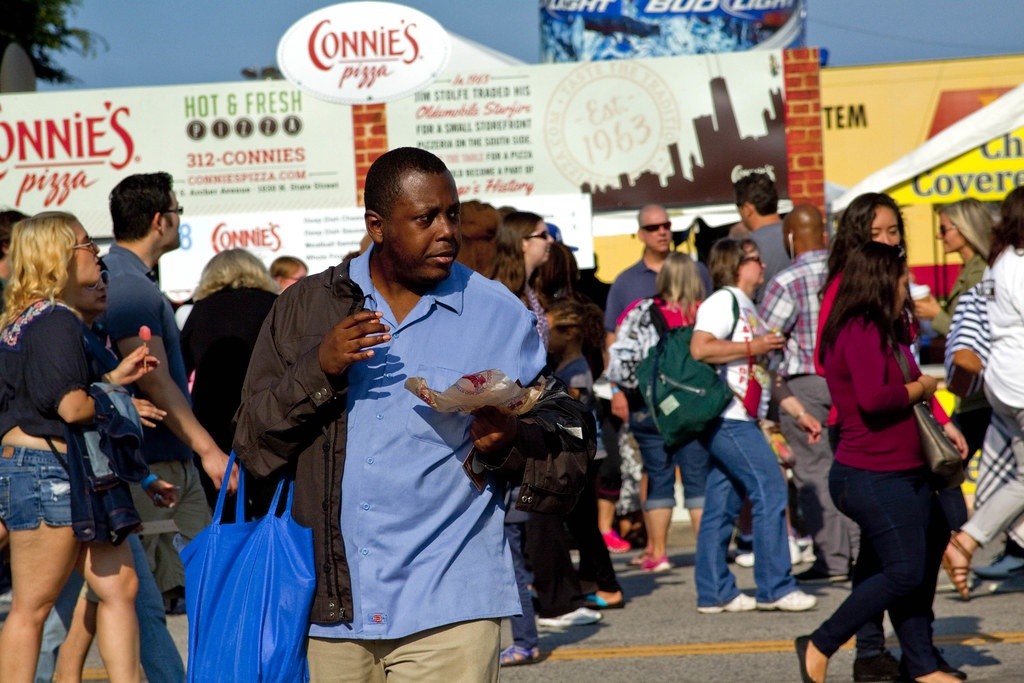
456, 173, 860, 667
918, 186, 1024, 599
0, 212, 28, 318
31, 257, 188, 683
234, 146, 547, 683
179, 248, 308, 522
813, 192, 969, 681
0, 211, 160, 683
54, 170, 239, 683
794, 242, 964, 683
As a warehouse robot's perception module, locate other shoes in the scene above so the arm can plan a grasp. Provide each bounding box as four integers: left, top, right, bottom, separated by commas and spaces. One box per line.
640, 556, 669, 573
736, 535, 803, 565
795, 635, 819, 683
627, 553, 651, 565
927, 646, 967, 680
797, 535, 816, 562
537, 608, 603, 628
901, 674, 922, 683
851, 650, 901, 683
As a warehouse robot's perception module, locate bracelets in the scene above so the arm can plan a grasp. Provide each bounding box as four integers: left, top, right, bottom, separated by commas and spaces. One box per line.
142, 475, 157, 487
918, 381, 925, 399
612, 387, 620, 393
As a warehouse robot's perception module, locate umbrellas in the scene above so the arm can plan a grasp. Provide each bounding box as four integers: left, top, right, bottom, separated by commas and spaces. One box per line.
831, 85, 1024, 213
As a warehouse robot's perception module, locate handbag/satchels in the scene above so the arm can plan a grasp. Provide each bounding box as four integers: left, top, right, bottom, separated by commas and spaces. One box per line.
945, 278, 996, 398
179, 444, 315, 683
635, 289, 739, 445
893, 341, 963, 471
743, 343, 762, 418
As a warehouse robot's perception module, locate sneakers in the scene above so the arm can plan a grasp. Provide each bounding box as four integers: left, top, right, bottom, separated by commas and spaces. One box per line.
756, 589, 818, 612
697, 592, 756, 615
600, 528, 631, 554
971, 550, 1024, 579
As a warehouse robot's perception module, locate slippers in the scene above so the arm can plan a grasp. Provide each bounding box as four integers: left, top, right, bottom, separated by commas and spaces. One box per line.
581, 594, 625, 611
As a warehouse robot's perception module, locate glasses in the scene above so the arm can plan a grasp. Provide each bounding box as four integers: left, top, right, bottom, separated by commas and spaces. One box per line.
895, 245, 908, 259
526, 230, 548, 240
640, 221, 671, 233
743, 256, 762, 263
74, 237, 96, 252
88, 271, 110, 289
940, 224, 957, 235
160, 206, 184, 216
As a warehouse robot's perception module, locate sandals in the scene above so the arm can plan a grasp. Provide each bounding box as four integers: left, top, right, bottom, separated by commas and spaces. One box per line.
499, 642, 541, 666
942, 533, 973, 600
